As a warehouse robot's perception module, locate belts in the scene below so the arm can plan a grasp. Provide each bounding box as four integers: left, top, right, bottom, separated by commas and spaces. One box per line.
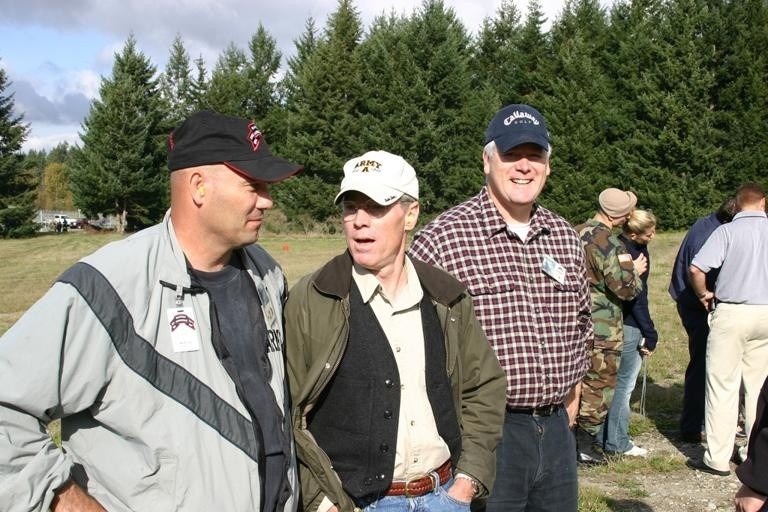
381, 459, 454, 496
507, 403, 564, 417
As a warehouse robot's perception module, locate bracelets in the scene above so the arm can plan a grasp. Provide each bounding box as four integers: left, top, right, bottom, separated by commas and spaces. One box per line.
696, 290, 708, 299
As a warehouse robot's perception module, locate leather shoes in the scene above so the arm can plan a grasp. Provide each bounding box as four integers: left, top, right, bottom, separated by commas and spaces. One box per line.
686, 456, 730, 476
736, 424, 747, 437
680, 426, 701, 444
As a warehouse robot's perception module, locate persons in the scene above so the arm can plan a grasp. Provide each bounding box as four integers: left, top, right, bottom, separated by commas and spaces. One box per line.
593, 208, 659, 459
689, 182, 768, 477
664, 197, 740, 445
0, 111, 302, 512
409, 102, 594, 512
730, 375, 767, 512
43, 215, 70, 234
275, 149, 508, 512
571, 187, 648, 466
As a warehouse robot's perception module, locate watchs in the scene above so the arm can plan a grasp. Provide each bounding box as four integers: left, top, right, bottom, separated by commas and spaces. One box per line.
454, 472, 480, 496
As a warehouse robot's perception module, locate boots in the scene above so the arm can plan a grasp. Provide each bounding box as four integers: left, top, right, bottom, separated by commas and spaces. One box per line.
575, 424, 608, 467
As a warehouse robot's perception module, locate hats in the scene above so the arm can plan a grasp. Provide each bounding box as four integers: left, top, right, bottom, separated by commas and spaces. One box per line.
597, 187, 637, 218
166, 112, 304, 183
485, 101, 549, 154
332, 151, 420, 208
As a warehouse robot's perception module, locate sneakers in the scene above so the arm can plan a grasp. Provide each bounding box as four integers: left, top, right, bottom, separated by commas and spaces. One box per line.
621, 443, 648, 456
